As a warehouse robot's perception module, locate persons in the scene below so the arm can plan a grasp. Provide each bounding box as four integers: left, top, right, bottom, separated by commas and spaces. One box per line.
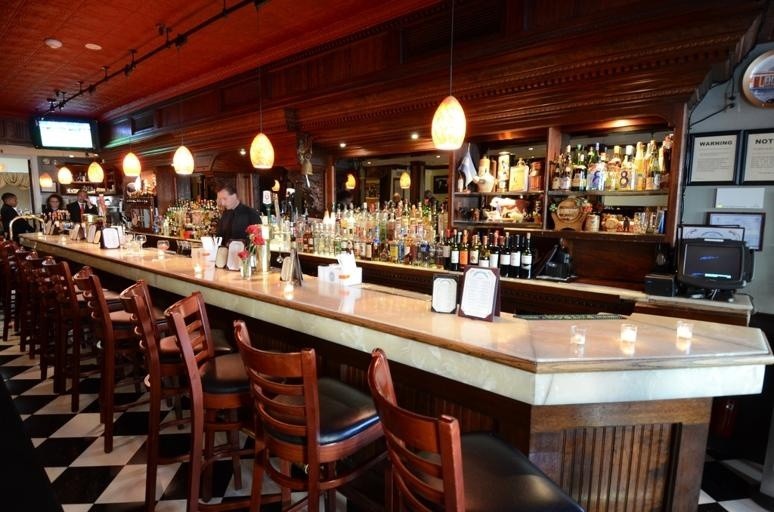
211, 187, 261, 245
64, 189, 99, 223
37, 194, 64, 231
0, 191, 37, 241
339, 184, 448, 212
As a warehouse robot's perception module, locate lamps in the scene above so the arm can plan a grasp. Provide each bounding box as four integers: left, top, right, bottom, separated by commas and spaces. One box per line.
344, 160, 413, 190
271, 178, 281, 192
249, 2, 274, 170
39, 3, 194, 189
430, 2, 466, 151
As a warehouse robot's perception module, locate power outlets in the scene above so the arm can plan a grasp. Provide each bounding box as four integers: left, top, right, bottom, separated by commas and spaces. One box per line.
725, 92, 741, 113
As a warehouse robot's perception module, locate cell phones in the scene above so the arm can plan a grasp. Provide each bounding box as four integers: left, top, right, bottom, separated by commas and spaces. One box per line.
83, 196, 89, 203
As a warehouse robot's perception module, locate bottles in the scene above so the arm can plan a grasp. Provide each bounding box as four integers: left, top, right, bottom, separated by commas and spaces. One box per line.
153, 198, 216, 239
268, 198, 443, 268
552, 140, 661, 191
443, 228, 533, 279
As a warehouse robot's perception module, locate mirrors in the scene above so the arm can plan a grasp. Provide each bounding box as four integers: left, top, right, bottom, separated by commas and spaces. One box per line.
333, 152, 449, 217
255, 166, 296, 217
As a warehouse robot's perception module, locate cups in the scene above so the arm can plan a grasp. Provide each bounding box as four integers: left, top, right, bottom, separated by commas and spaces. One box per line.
619, 324, 638, 342
570, 346, 585, 360
620, 342, 636, 357
633, 211, 648, 235
190, 247, 214, 275
675, 320, 693, 339
569, 325, 588, 345
675, 339, 693, 355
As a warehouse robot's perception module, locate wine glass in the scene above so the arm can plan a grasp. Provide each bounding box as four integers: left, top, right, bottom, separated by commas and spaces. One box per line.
155, 239, 170, 257
135, 234, 146, 253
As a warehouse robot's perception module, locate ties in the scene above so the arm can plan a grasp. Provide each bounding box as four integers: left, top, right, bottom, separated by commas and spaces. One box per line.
81, 204, 85, 214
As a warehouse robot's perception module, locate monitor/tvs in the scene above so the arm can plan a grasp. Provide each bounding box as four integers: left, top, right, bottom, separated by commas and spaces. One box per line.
30, 113, 102, 153
679, 239, 753, 302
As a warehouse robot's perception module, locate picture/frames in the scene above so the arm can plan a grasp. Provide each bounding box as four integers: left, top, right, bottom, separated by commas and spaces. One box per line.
742, 128, 774, 187
686, 129, 745, 188
433, 175, 448, 194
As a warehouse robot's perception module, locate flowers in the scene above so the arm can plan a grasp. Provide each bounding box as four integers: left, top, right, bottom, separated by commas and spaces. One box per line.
236, 226, 264, 260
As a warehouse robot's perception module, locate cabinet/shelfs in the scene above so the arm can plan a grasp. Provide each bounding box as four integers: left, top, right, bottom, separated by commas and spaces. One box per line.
55, 162, 123, 196
452, 123, 689, 251
